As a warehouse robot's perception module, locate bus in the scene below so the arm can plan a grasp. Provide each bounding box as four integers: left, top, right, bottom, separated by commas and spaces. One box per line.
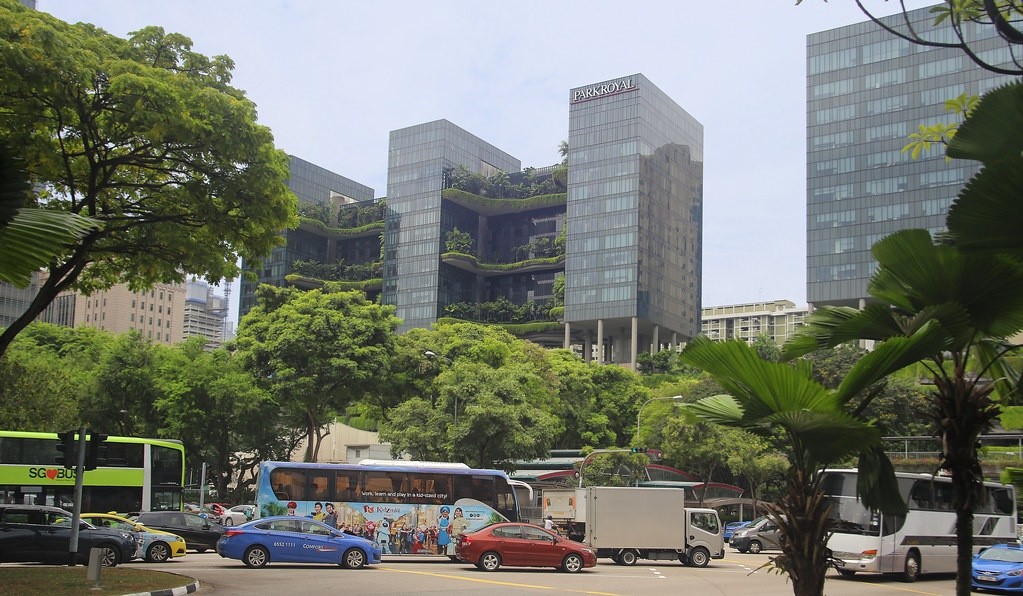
253, 460, 534, 564
812, 469, 1018, 582
0, 431, 186, 529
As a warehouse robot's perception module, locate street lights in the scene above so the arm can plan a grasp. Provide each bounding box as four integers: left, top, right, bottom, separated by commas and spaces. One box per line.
637, 396, 683, 441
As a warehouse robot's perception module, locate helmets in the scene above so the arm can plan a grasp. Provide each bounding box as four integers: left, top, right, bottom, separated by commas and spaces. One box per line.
548, 515, 552, 520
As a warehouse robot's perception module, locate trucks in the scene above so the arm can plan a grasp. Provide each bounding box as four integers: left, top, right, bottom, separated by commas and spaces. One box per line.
585, 485, 724, 567
542, 488, 586, 543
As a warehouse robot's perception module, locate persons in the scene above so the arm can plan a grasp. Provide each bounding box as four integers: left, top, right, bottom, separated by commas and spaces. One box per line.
286, 502, 298, 516
312, 502, 338, 533
545, 514, 557, 534
437, 506, 469, 555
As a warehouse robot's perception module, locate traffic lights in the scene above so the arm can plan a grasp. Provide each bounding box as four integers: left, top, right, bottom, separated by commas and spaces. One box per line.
631, 447, 648, 453
86, 433, 108, 469
55, 430, 74, 469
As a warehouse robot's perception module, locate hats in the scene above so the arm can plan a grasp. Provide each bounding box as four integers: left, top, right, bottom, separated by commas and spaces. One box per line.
288, 502, 297, 509
440, 506, 450, 513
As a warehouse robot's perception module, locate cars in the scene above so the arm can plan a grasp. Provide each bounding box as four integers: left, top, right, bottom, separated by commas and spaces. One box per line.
455, 522, 597, 574
125, 512, 223, 553
972, 543, 1023, 596
172, 503, 255, 527
0, 505, 138, 567
54, 513, 186, 562
724, 522, 750, 544
216, 515, 382, 569
728, 515, 788, 554
